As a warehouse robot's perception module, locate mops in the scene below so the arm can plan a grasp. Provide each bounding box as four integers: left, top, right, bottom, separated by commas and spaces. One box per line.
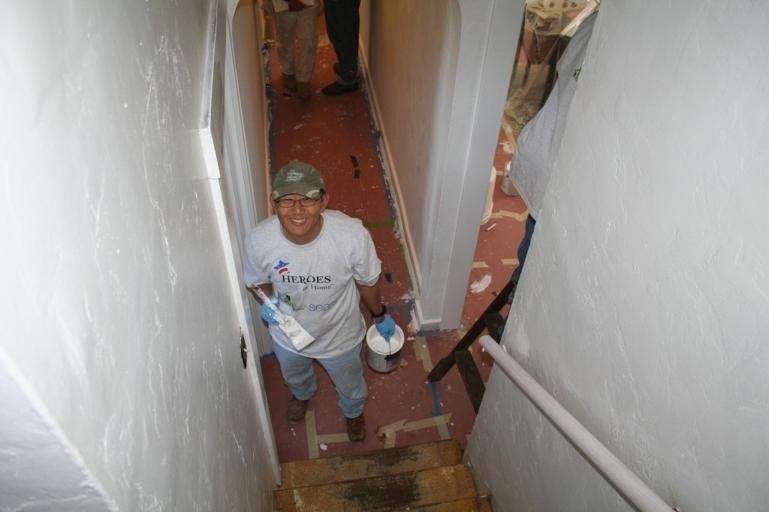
505, 37, 560, 126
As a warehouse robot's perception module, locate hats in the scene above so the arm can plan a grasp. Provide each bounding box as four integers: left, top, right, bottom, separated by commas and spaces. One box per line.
271, 161, 325, 200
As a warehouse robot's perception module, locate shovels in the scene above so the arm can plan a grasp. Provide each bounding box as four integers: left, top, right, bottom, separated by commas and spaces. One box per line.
246, 279, 316, 352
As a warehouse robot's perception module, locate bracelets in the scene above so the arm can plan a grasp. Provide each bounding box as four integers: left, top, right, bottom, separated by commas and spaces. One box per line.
369, 304, 387, 318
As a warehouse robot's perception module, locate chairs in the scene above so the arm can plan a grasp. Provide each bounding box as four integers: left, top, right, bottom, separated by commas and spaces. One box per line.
522, 27, 568, 108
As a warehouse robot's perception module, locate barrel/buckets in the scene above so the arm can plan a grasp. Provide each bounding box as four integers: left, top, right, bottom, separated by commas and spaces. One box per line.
481, 166, 498, 232
500, 162, 520, 197
366, 324, 406, 374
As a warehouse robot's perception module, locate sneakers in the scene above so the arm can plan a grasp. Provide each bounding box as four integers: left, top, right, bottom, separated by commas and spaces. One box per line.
347, 412, 366, 442
287, 395, 309, 424
281, 72, 296, 90
296, 81, 311, 100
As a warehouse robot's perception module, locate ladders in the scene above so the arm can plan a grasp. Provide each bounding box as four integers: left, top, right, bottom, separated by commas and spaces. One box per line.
427, 278, 516, 415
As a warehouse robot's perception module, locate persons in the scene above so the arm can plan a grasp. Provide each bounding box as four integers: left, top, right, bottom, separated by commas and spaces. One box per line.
241, 159, 398, 446
322, 1, 364, 97
261, 1, 327, 102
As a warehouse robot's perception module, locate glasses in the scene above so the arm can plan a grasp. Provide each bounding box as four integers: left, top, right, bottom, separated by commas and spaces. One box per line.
274, 197, 317, 208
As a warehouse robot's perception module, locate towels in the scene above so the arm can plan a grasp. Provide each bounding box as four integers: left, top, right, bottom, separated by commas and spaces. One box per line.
558, 0, 600, 38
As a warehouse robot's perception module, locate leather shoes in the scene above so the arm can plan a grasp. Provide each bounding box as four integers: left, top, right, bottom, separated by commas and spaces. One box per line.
333, 63, 342, 76
322, 81, 358, 96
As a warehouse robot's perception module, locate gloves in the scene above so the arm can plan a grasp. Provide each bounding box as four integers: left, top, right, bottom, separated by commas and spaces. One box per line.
373, 314, 396, 342
259, 296, 281, 325
